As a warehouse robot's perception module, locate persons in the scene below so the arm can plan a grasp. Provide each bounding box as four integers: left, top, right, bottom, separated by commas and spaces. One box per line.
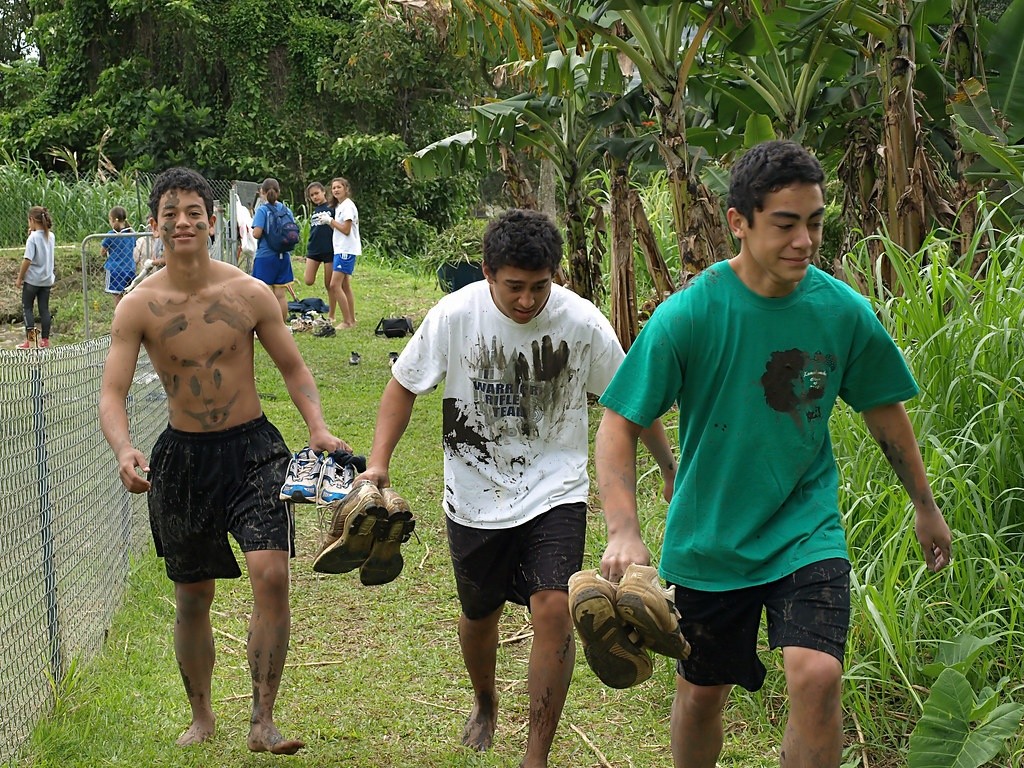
348, 208, 678, 768
99, 203, 167, 307
590, 140, 955, 768
98, 165, 355, 755
12, 204, 55, 349
251, 178, 363, 331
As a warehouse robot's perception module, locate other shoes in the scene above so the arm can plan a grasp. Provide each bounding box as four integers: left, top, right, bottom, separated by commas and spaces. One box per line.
38, 338, 48, 349
15, 341, 29, 350
313, 325, 336, 338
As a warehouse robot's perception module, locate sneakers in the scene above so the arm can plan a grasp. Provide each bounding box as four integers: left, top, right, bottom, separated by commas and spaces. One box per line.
349, 351, 359, 365
617, 563, 692, 660
316, 451, 366, 506
313, 479, 389, 575
568, 568, 653, 689
388, 351, 399, 367
360, 488, 416, 586
278, 447, 323, 502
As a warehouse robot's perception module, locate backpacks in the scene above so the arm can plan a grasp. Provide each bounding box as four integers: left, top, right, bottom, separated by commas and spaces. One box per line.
262, 204, 300, 252
374, 315, 415, 337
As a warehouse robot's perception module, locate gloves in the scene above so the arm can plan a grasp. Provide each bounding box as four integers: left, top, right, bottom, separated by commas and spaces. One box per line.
318, 211, 333, 226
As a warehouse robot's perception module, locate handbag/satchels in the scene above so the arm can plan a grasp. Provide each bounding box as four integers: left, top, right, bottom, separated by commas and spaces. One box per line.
235, 195, 258, 254
